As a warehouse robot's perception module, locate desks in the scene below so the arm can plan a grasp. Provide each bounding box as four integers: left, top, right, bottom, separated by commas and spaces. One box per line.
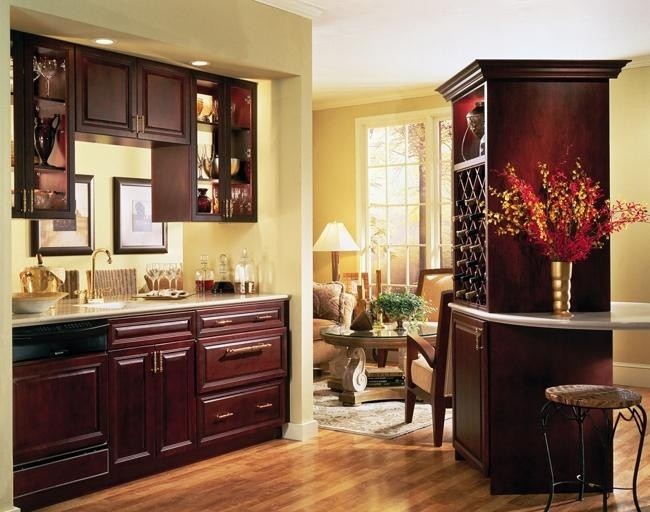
320, 322, 440, 407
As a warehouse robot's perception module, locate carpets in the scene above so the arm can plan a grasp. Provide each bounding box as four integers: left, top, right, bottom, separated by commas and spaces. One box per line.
313, 371, 454, 441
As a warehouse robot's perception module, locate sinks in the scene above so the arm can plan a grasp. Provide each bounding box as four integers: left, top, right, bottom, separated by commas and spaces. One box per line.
72, 299, 179, 311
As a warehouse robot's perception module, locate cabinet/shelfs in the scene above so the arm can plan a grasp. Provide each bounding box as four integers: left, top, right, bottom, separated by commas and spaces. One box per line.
448, 311, 615, 496
111, 306, 197, 489
434, 57, 632, 315
197, 296, 294, 462
73, 39, 193, 148
150, 67, 259, 224
10, 28, 77, 221
13, 317, 110, 512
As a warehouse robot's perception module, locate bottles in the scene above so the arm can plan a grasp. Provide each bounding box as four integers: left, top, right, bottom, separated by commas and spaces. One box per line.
465, 101, 485, 140
347, 300, 373, 331
232, 248, 258, 294
33, 113, 60, 169
193, 255, 215, 294
372, 304, 387, 331
211, 253, 234, 293
31, 189, 50, 210
196, 188, 212, 214
45, 189, 66, 212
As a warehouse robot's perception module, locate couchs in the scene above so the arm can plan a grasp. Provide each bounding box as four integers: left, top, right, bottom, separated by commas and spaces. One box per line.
313, 282, 358, 380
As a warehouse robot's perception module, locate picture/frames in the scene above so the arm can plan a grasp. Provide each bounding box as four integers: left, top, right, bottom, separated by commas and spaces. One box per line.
29, 172, 97, 258
112, 175, 168, 256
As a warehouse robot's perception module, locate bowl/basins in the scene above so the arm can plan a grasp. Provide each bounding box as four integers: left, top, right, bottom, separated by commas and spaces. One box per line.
12, 292, 68, 315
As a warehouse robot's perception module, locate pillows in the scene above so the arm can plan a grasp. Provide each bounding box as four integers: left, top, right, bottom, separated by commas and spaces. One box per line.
313, 280, 346, 325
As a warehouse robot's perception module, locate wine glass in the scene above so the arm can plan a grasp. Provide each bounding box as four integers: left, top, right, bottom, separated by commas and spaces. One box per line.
32, 62, 41, 82
145, 260, 183, 297
197, 96, 241, 179
212, 185, 250, 217
36, 59, 57, 100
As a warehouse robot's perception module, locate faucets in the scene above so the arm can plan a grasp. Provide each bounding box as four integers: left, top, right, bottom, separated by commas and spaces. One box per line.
88, 248, 112, 300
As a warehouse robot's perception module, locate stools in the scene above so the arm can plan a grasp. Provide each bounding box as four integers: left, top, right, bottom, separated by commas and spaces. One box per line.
535, 381, 648, 512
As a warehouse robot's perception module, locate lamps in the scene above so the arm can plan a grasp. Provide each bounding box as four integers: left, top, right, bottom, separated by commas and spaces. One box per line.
313, 221, 361, 282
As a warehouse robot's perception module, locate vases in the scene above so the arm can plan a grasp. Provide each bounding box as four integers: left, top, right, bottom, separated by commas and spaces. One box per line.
550, 261, 576, 320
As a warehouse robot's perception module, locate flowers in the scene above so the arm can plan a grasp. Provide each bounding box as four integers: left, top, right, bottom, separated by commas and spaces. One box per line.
475, 157, 650, 263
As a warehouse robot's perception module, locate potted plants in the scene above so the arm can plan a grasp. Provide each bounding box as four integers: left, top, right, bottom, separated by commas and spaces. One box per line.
367, 291, 439, 332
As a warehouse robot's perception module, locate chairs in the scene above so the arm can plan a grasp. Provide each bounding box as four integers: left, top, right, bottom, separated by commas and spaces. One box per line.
411, 267, 454, 356
403, 288, 458, 447
